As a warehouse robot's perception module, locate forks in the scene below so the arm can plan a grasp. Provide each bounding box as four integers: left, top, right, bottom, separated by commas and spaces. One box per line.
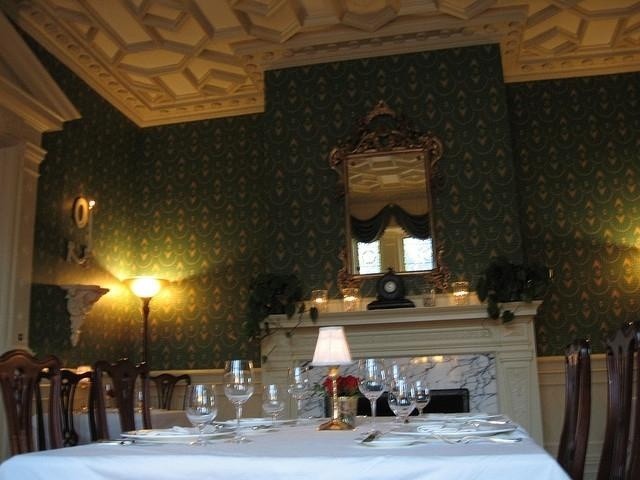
433, 431, 522, 446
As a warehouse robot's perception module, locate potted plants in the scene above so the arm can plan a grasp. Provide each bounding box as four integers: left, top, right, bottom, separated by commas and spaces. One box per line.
476, 252, 539, 321
244, 263, 319, 364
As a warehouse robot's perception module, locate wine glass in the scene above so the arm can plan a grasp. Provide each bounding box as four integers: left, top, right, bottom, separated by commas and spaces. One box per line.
357, 358, 385, 436
385, 378, 417, 431
185, 384, 219, 447
412, 381, 432, 422
222, 358, 254, 447
262, 384, 286, 432
381, 362, 412, 423
287, 366, 314, 428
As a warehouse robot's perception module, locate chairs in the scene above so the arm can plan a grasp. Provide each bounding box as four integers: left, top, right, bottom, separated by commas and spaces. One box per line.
0, 349, 66, 466
151, 372, 192, 410
555, 337, 594, 480
93, 358, 155, 442
34, 369, 97, 452
594, 323, 640, 480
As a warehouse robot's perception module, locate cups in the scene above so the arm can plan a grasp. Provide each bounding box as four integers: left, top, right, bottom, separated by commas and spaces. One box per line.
312, 288, 330, 311
340, 287, 363, 312
421, 286, 436, 308
451, 280, 470, 306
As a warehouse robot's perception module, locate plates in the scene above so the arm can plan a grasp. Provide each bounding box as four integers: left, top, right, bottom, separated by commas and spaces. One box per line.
353, 430, 423, 448
213, 418, 286, 429
418, 410, 516, 435
119, 428, 237, 443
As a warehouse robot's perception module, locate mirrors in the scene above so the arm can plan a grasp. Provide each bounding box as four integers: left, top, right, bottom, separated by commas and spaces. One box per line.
329, 98, 442, 284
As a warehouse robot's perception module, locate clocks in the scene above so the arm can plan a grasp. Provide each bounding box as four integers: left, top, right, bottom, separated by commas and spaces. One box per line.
366, 267, 416, 309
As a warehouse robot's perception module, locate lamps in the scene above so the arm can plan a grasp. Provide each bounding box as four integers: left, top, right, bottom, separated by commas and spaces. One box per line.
129, 274, 159, 368
65, 197, 96, 267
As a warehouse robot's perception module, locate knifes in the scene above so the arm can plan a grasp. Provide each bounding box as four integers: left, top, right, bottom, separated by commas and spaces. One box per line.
361, 430, 380, 443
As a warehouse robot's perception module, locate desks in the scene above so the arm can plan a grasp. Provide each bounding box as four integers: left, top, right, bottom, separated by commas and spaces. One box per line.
60, 409, 195, 441
0, 416, 569, 479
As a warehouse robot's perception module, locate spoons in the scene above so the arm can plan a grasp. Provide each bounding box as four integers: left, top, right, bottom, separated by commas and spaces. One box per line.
93, 440, 138, 449
